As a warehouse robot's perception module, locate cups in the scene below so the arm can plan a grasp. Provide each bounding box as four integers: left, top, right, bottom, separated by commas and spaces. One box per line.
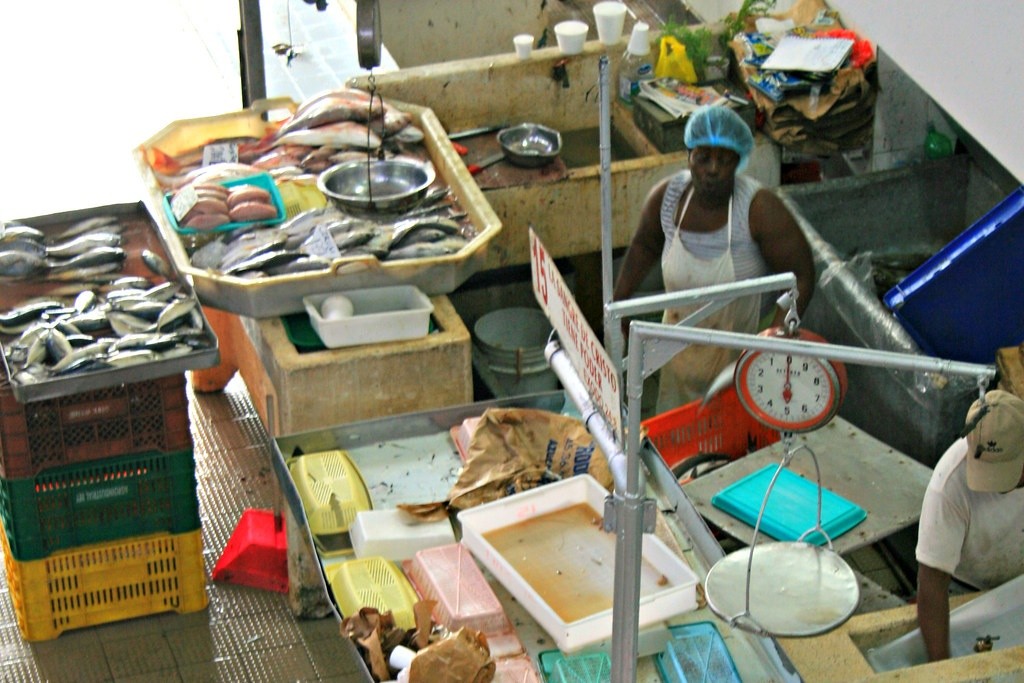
627, 21, 652, 55
592, 1, 627, 45
513, 34, 534, 59
554, 20, 589, 54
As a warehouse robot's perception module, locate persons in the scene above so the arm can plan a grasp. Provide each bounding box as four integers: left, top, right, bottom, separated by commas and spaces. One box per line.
916, 389, 1024, 662
613, 106, 815, 482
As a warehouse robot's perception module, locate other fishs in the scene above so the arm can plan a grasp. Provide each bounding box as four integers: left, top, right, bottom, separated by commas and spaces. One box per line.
1, 215, 206, 387
148, 88, 470, 276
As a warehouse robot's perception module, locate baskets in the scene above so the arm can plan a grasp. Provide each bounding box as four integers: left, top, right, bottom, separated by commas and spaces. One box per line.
324, 556, 420, 631
285, 449, 373, 534
162, 172, 288, 233
653, 622, 743, 683
537, 646, 611, 683
402, 543, 512, 636
276, 178, 327, 221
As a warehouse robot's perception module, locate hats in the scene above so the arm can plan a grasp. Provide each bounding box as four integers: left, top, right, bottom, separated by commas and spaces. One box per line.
963, 389, 1024, 492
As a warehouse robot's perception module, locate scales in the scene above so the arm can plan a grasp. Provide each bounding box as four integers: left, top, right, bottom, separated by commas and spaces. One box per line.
701, 280, 877, 638
314, 0, 436, 226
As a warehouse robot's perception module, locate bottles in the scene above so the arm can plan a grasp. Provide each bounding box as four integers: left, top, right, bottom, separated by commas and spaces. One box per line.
923, 124, 952, 159
616, 50, 657, 111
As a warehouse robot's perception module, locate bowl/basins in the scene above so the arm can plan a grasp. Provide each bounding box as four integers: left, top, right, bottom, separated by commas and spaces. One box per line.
497, 122, 562, 167
317, 156, 436, 217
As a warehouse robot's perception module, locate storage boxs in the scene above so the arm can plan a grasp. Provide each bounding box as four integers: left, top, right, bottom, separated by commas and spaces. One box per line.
456, 474, 697, 655
0, 367, 213, 643
303, 284, 434, 349
348, 508, 455, 559
640, 385, 781, 469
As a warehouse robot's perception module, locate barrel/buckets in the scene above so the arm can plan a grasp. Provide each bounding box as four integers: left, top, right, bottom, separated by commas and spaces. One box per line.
475, 306, 560, 410
